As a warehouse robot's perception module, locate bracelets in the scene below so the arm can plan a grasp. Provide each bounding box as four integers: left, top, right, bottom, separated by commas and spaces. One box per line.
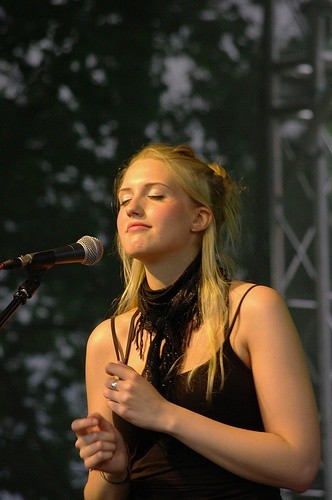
99, 469, 129, 485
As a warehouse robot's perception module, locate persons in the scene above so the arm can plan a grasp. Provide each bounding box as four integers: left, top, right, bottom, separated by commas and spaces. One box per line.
71, 145, 321, 500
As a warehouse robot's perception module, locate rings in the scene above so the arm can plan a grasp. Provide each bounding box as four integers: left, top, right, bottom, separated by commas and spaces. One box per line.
111, 379, 118, 390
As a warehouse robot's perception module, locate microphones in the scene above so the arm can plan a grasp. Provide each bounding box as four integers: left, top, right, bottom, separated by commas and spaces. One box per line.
0, 235, 103, 271
